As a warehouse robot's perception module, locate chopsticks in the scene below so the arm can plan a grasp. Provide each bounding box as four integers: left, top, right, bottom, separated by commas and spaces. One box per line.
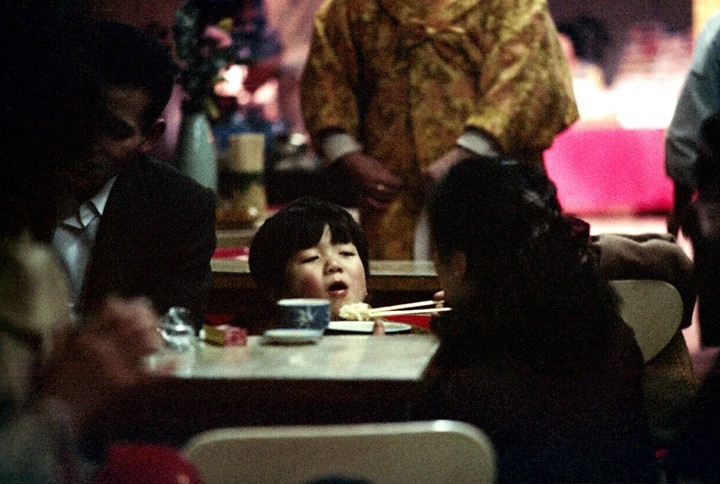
366, 298, 452, 318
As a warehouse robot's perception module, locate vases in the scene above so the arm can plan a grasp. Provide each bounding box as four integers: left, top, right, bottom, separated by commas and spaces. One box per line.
175, 112, 217, 196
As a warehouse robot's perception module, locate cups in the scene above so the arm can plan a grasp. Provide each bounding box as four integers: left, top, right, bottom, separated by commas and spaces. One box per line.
276, 298, 331, 333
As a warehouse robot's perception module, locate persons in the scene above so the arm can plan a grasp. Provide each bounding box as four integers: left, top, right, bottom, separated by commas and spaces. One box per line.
247, 197, 370, 313
663, 17, 720, 350
0, 19, 217, 302
408, 156, 659, 484
0, 290, 160, 484
300, 0, 578, 257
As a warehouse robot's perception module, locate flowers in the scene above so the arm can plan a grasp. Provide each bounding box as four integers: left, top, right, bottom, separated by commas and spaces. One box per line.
173, 0, 284, 114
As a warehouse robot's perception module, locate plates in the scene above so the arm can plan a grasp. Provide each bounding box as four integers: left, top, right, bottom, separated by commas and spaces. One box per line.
264, 327, 323, 344
325, 320, 411, 333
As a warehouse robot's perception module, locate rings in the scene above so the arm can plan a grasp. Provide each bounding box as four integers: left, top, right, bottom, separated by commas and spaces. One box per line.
376, 185, 383, 192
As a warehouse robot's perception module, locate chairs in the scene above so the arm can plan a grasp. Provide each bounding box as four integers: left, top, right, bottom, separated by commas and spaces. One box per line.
610, 279, 685, 365
177, 418, 500, 484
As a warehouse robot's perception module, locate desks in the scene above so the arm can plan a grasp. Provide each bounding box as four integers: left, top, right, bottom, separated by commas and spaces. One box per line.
124, 333, 441, 452
209, 253, 441, 333
